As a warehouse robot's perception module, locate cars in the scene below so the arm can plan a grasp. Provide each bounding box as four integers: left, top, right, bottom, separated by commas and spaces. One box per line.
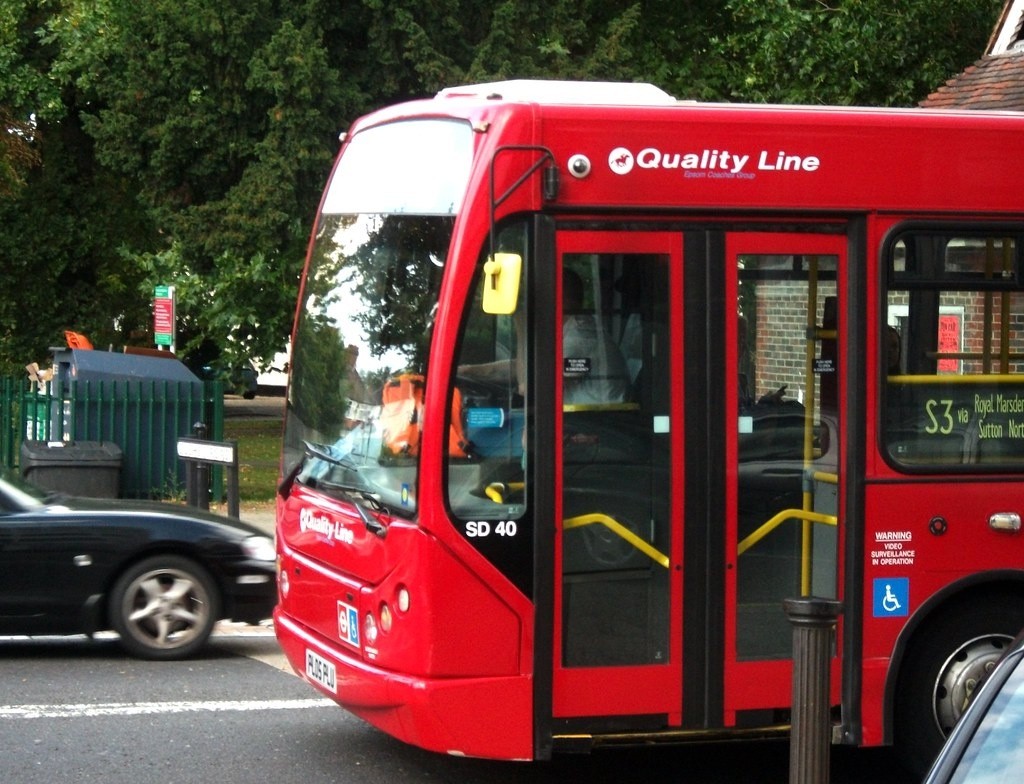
921, 635, 1024, 784
0, 467, 279, 662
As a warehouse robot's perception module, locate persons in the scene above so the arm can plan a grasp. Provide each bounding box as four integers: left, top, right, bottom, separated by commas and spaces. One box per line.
454, 266, 634, 405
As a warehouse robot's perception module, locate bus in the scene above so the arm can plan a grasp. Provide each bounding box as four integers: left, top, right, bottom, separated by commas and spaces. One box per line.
271, 80, 1024, 774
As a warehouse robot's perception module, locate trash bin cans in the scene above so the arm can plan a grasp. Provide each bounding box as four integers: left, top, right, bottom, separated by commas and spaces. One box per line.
19, 440, 123, 498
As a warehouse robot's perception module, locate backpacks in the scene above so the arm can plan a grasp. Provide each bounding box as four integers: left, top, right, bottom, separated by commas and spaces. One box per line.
382, 373, 475, 464
